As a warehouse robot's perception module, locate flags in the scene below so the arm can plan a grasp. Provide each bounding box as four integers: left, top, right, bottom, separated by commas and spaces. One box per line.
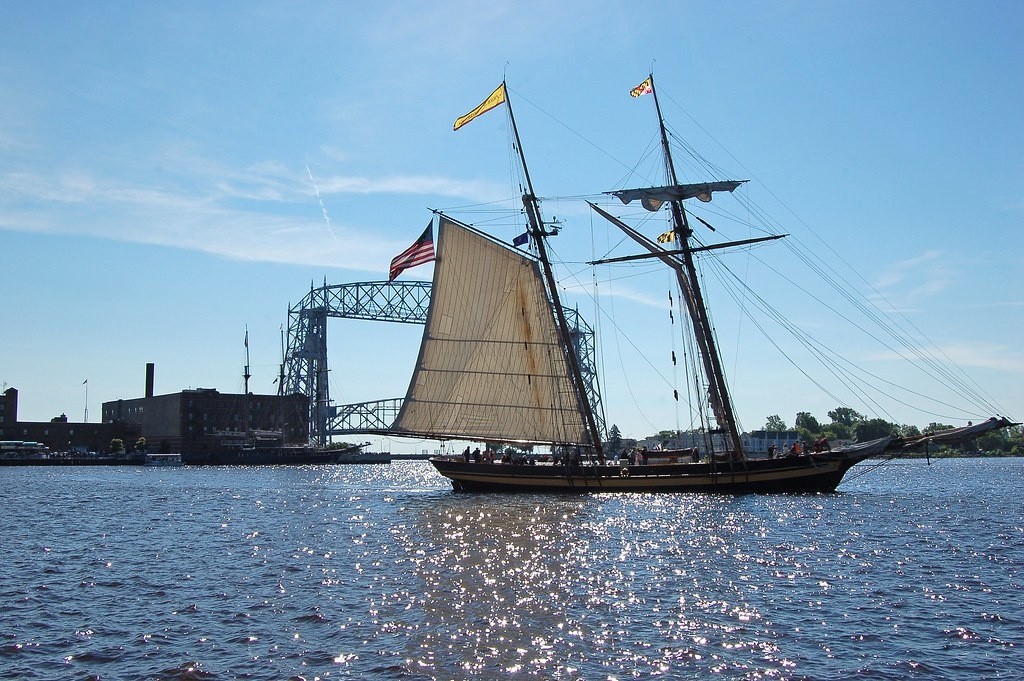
629, 77, 652, 97
245, 330, 249, 348
452, 81, 506, 131
657, 230, 675, 244
389, 219, 437, 283
83, 379, 88, 384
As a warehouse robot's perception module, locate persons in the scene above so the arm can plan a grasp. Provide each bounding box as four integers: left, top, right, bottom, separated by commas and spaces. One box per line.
690, 446, 700, 464
620, 445, 649, 466
767, 437, 831, 458
562, 451, 570, 467
538, 453, 550, 463
463, 445, 536, 466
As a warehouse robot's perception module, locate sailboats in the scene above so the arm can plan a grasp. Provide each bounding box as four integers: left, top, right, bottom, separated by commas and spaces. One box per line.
386, 59, 1024, 492
180, 323, 373, 464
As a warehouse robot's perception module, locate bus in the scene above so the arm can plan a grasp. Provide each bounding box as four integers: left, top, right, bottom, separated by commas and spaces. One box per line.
0, 441, 49, 459
